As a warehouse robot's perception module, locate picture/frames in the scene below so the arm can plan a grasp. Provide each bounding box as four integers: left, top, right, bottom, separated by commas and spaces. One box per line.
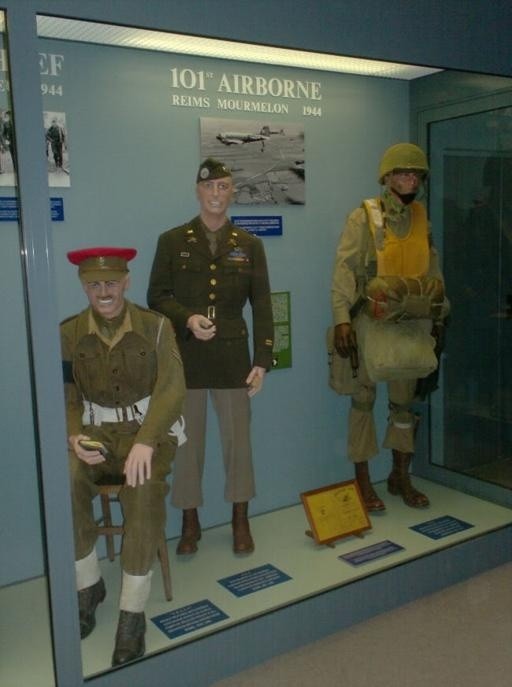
300, 479, 371, 544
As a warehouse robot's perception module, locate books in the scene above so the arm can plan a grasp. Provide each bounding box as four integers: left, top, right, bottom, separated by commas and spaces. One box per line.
78, 439, 111, 456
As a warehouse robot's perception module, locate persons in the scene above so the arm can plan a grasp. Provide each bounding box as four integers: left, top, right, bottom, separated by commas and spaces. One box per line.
59, 246, 188, 666
326, 145, 450, 512
48, 117, 66, 167
146, 151, 278, 553
3, 110, 16, 168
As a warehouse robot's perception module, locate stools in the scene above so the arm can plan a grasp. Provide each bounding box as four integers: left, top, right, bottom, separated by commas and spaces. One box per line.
88, 473, 174, 604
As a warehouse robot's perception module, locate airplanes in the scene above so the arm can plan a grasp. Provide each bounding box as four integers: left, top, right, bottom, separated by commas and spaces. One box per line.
216, 125, 286, 146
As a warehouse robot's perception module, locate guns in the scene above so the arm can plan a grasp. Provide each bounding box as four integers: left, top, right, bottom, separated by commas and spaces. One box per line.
347, 329, 360, 379
